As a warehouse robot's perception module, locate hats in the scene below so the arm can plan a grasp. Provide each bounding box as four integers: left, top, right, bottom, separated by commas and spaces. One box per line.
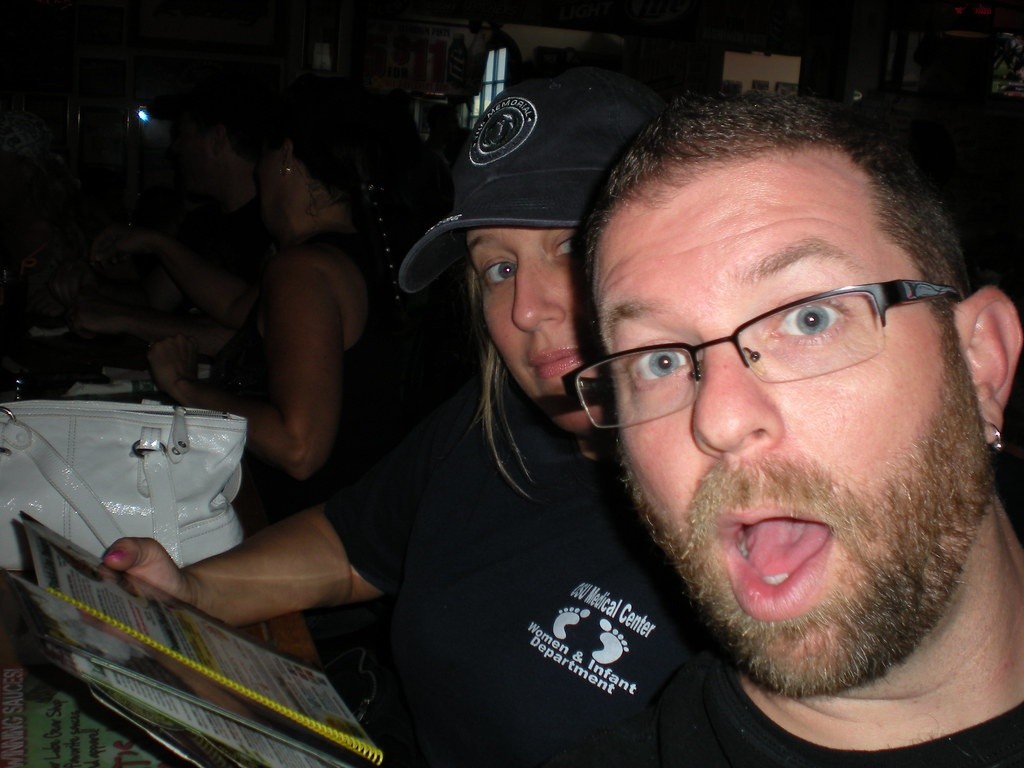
397, 69, 643, 295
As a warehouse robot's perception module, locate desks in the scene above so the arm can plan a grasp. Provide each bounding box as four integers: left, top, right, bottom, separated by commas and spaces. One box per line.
2, 527, 331, 768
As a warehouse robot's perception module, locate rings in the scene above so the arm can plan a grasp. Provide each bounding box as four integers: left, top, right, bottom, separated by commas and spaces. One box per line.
147, 343, 154, 351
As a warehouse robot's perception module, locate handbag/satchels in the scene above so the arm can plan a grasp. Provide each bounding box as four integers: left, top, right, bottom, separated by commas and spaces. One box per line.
0, 394, 248, 573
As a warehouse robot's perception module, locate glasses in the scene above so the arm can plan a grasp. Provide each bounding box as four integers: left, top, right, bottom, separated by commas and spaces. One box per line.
561, 279, 963, 429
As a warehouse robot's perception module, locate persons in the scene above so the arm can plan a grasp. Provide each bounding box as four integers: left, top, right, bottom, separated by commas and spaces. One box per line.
540, 88, 1024, 768
103, 63, 719, 768
44, 60, 284, 359
0, 110, 91, 320
90, 73, 443, 643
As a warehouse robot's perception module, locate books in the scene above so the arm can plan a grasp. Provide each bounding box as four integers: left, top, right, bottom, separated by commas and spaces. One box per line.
0, 510, 391, 768
